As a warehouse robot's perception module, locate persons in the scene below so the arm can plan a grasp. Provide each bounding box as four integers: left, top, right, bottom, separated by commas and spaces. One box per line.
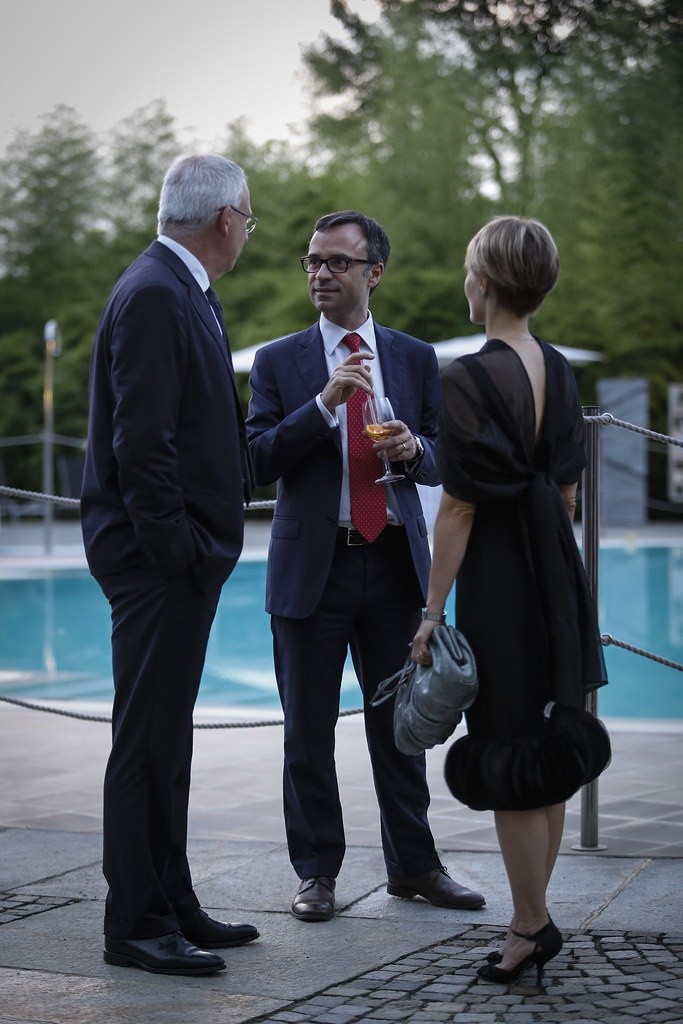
246, 207, 490, 920
410, 214, 612, 986
80, 152, 263, 974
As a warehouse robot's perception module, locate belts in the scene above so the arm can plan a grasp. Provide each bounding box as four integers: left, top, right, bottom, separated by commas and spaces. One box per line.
337, 526, 406, 546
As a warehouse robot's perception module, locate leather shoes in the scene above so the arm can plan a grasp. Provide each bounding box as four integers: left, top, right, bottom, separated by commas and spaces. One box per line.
387, 866, 486, 910
102, 930, 227, 977
290, 875, 336, 920
180, 908, 261, 949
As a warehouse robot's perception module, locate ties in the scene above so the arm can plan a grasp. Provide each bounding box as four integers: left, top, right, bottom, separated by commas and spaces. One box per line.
205, 286, 252, 508
342, 333, 388, 544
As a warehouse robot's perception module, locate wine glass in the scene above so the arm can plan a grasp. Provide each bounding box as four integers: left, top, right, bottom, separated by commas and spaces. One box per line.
362, 397, 405, 483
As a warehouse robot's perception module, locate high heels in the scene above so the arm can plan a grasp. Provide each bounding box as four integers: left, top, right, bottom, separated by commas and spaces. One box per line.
476, 912, 563, 987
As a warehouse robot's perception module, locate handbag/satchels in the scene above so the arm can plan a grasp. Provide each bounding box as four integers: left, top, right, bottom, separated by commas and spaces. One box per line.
369, 624, 480, 757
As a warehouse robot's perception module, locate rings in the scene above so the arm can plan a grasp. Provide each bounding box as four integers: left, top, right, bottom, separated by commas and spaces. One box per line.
402, 443, 407, 450
416, 652, 422, 657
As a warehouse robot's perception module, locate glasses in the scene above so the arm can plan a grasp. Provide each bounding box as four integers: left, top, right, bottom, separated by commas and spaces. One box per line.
214, 204, 258, 234
299, 255, 374, 274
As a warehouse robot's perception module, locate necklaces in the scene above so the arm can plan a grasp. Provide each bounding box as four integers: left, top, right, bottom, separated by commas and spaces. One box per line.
501, 337, 536, 342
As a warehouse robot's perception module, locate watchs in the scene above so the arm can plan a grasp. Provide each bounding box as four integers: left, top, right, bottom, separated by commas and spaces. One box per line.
421, 607, 447, 622
413, 436, 424, 461
